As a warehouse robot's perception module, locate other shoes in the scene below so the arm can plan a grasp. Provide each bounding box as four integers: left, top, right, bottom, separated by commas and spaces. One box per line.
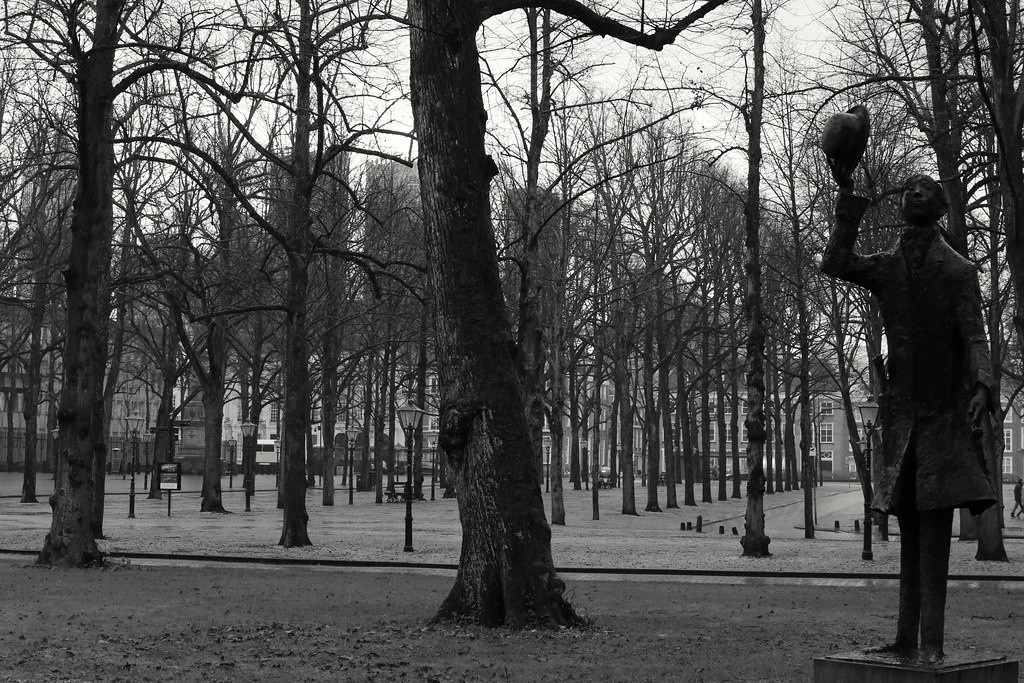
1011, 512, 1014, 517
1017, 514, 1020, 519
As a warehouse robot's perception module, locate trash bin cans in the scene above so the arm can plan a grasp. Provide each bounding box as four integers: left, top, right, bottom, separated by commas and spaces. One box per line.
355, 473, 365, 492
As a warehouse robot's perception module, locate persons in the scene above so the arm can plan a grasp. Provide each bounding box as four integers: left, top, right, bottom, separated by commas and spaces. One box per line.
1011, 478, 1024, 521
819, 105, 998, 665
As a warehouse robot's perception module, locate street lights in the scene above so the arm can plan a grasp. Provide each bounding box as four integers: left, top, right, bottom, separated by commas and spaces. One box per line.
274, 437, 282, 486
124, 410, 146, 519
396, 400, 425, 551
240, 418, 258, 513
427, 431, 439, 500
580, 437, 590, 491
345, 423, 360, 504
616, 441, 622, 488
140, 429, 151, 490
857, 395, 879, 560
228, 437, 237, 489
50, 425, 61, 490
543, 436, 552, 492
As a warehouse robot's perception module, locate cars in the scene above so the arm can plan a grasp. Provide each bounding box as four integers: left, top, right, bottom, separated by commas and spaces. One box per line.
725, 470, 750, 480
598, 465, 611, 477
